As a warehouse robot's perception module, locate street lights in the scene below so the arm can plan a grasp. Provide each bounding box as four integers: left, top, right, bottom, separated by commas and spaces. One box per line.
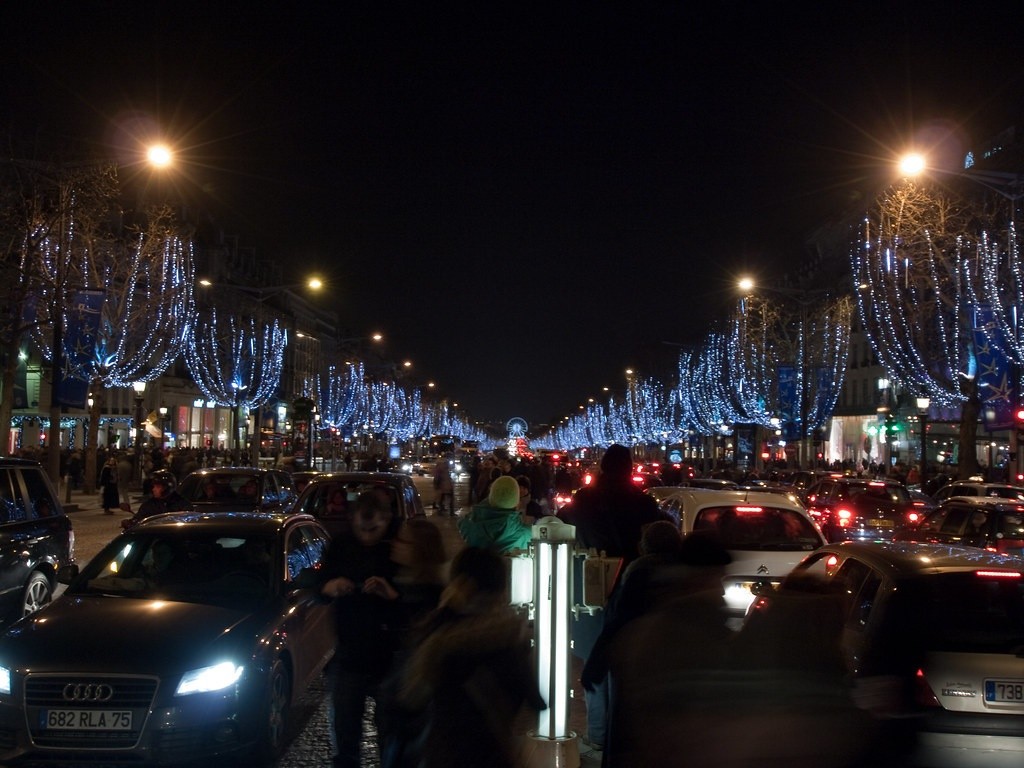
884, 141, 1024, 488
0, 133, 183, 499
734, 271, 870, 472
198, 274, 325, 469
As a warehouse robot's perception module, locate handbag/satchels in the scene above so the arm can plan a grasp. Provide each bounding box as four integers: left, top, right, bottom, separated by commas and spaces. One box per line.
434, 476, 441, 487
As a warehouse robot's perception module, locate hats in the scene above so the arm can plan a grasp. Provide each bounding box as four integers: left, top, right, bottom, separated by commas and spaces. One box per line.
601, 444, 632, 474
489, 476, 520, 510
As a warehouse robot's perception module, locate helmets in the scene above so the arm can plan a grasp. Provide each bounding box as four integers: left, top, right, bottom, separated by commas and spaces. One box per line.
152, 471, 177, 495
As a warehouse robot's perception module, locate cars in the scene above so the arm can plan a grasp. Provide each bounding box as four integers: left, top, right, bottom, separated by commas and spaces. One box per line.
292, 471, 431, 551
172, 465, 300, 516
417, 456, 438, 477
728, 538, 1024, 720
630, 461, 1024, 559
658, 490, 841, 620
0, 512, 339, 768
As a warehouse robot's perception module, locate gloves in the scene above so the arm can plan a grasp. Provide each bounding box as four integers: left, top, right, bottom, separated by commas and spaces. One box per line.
580, 665, 606, 694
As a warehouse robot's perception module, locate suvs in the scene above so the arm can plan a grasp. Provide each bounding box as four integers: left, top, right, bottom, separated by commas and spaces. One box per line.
0, 456, 77, 624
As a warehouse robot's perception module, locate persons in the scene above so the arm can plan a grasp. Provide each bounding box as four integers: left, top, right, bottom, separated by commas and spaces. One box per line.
555, 444, 677, 751
681, 569, 925, 768
345, 452, 351, 472
369, 486, 394, 516
96, 444, 136, 491
979, 459, 1008, 479
455, 474, 544, 554
121, 472, 177, 530
431, 451, 458, 517
99, 456, 119, 515
234, 479, 257, 512
215, 444, 249, 467
197, 480, 221, 502
465, 447, 555, 506
580, 521, 738, 768
325, 488, 348, 515
310, 487, 537, 767
296, 480, 307, 493
142, 446, 213, 494
33, 497, 52, 517
360, 455, 389, 472
10, 444, 86, 493
659, 455, 803, 486
807, 458, 952, 483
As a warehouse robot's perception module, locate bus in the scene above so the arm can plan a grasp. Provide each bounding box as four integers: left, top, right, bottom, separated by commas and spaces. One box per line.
428, 434, 462, 459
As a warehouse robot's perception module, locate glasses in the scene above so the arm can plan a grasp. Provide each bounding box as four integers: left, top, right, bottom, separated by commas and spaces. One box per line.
392, 536, 416, 546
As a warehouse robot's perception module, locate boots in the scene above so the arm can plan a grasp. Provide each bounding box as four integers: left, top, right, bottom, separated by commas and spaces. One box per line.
450, 507, 458, 517
